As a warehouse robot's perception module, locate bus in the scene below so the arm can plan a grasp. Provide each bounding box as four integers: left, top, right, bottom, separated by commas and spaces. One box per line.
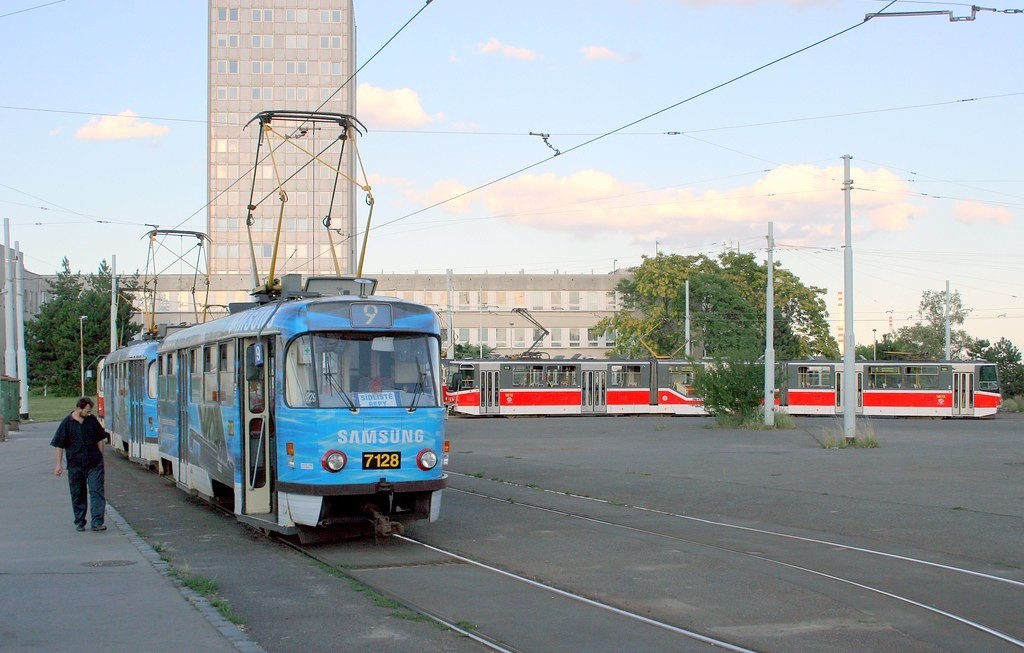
96, 273, 461, 546
448, 358, 1004, 417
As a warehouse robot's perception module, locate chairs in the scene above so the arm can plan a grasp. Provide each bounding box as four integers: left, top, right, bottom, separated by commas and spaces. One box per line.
249, 402, 274, 438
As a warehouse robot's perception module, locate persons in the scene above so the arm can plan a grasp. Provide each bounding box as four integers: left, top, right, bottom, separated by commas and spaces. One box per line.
50, 397, 108, 532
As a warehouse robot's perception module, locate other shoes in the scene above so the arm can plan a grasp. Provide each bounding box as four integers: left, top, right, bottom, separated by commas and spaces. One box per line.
76, 524, 85, 530
91, 524, 107, 530
